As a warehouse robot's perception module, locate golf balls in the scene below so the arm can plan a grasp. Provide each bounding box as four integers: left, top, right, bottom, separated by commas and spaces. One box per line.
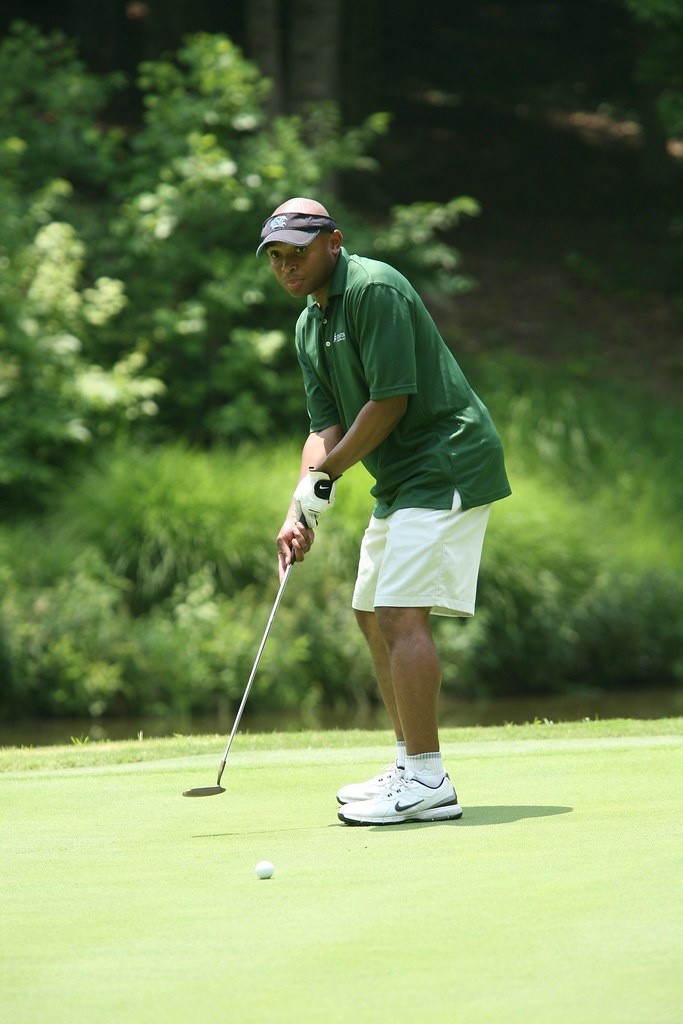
255, 860, 275, 879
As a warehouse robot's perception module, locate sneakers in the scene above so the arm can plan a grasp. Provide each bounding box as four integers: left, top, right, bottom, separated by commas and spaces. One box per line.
336, 758, 463, 826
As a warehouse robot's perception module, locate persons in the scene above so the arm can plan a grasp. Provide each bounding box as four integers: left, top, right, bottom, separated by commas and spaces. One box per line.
255, 194, 513, 826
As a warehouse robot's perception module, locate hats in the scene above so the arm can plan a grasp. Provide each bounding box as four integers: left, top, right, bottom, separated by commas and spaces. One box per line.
256, 197, 337, 259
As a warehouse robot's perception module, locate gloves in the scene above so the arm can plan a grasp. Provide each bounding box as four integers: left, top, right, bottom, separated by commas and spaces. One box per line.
293, 467, 343, 528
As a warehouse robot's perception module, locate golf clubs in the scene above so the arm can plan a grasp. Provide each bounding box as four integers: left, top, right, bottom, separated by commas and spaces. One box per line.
181, 512, 309, 799
